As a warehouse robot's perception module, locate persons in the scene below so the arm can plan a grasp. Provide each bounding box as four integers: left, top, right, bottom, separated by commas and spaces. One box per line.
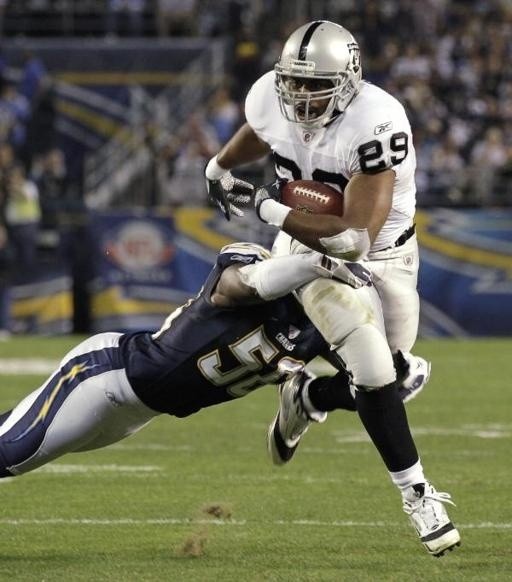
0, 41, 74, 285
104, 0, 199, 40
64, 210, 98, 336
0, 223, 434, 481
158, 1, 511, 213
203, 19, 462, 556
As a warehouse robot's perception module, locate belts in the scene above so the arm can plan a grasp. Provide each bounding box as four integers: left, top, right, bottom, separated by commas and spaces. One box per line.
379, 223, 416, 251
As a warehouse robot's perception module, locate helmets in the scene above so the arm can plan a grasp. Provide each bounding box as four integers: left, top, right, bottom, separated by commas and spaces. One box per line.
274, 19, 362, 124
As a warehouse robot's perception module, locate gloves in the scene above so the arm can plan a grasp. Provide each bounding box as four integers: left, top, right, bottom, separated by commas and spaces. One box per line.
203, 158, 255, 221
312, 253, 374, 289
254, 178, 288, 224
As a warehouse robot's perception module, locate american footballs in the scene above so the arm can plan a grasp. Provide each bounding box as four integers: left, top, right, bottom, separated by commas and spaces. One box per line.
280, 180, 343, 220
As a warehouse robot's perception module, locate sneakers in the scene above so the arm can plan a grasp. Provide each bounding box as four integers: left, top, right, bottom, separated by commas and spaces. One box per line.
402, 482, 461, 558
268, 371, 328, 468
